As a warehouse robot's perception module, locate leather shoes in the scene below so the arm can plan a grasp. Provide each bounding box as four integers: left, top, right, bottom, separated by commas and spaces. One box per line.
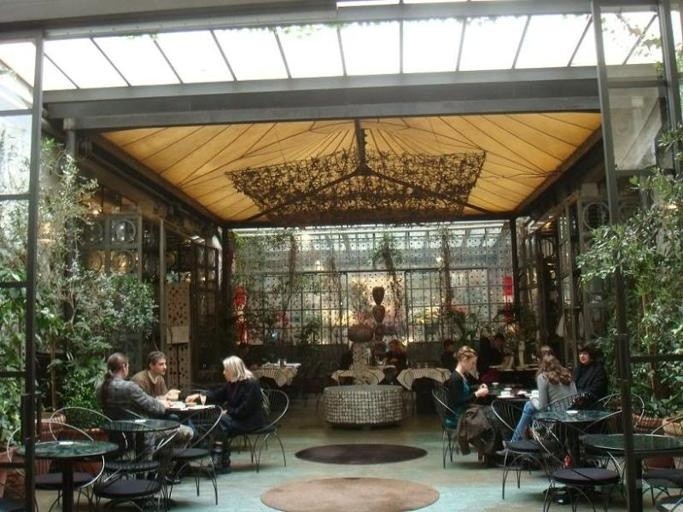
495, 448, 510, 456
163, 475, 181, 483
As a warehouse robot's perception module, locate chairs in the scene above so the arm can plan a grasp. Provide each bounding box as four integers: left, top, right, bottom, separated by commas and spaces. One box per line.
6, 388, 291, 512
429, 366, 683, 512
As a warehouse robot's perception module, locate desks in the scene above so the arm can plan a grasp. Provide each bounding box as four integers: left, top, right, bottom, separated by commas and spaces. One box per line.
396, 367, 452, 416
331, 365, 397, 387
320, 384, 407, 431
251, 362, 303, 387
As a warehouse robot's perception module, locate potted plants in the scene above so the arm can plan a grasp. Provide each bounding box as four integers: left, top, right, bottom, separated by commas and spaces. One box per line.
573, 122, 683, 470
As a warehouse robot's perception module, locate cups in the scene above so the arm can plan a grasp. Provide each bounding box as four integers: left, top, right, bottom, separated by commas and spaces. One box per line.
523, 394, 531, 398
566, 410, 578, 416
492, 382, 499, 387
504, 387, 512, 395
501, 391, 511, 397
531, 390, 539, 396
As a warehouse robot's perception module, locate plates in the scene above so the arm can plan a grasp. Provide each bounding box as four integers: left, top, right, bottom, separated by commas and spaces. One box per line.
496, 396, 515, 399
185, 402, 197, 407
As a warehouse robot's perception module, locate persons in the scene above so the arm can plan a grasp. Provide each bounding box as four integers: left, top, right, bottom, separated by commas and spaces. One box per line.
238, 333, 514, 382
130, 351, 198, 448
496, 345, 577, 457
185, 355, 263, 474
448, 345, 515, 464
96, 352, 194, 483
573, 344, 608, 409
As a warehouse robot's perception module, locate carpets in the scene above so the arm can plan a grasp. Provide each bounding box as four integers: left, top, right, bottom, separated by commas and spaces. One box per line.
293, 442, 429, 466
259, 475, 441, 512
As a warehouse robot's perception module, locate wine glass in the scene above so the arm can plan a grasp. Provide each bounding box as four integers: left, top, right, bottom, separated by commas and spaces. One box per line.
200, 391, 207, 408
